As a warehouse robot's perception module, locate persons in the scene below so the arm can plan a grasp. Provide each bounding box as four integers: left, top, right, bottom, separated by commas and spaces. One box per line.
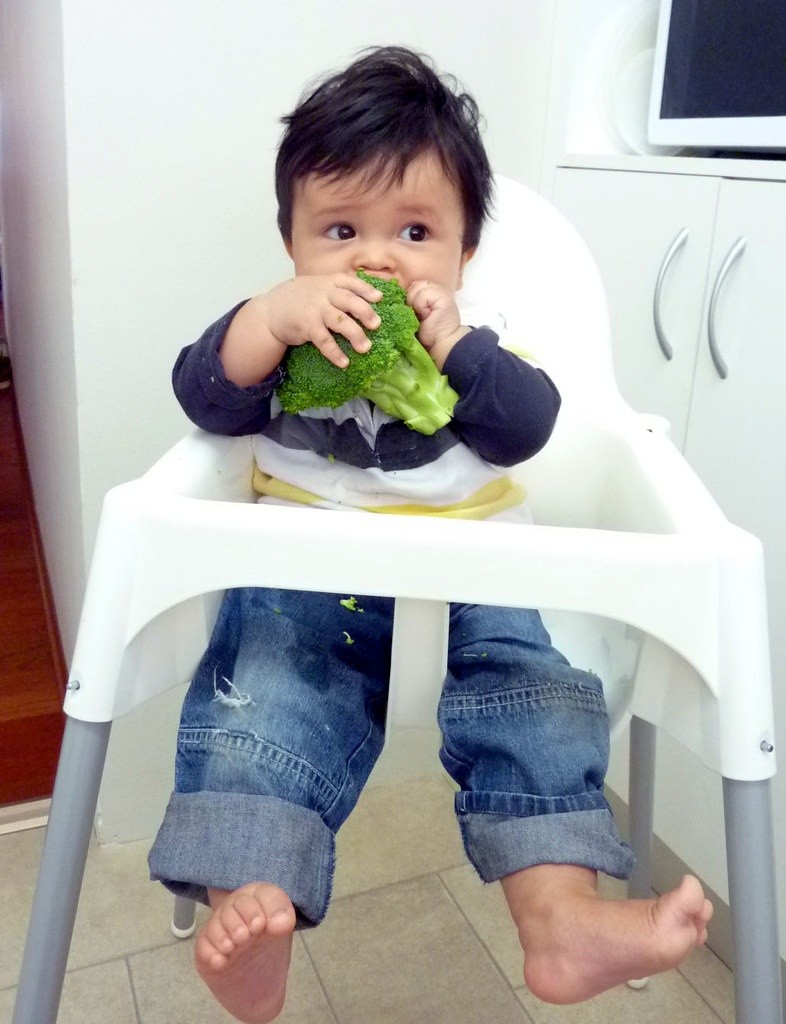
142, 44, 716, 1024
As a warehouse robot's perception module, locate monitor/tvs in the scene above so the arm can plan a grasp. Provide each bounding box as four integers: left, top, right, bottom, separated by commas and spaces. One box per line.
647, 0, 786, 154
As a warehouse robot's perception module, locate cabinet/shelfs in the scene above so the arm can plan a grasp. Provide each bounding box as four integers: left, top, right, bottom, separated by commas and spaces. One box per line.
584, 170, 786, 724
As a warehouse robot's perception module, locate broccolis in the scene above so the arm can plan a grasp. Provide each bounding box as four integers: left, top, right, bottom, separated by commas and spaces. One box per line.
275, 268, 459, 435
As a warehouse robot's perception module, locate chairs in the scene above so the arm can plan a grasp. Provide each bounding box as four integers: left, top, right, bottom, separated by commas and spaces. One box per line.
5, 167, 785, 1023
